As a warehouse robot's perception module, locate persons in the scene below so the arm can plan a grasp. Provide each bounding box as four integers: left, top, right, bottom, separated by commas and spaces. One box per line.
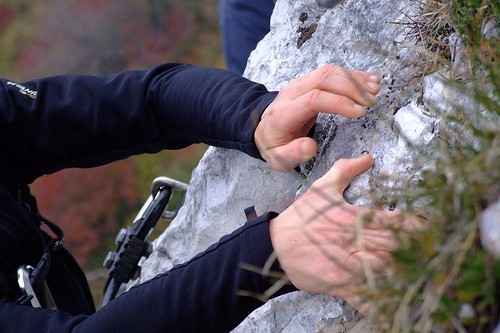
0, 63, 429, 333
